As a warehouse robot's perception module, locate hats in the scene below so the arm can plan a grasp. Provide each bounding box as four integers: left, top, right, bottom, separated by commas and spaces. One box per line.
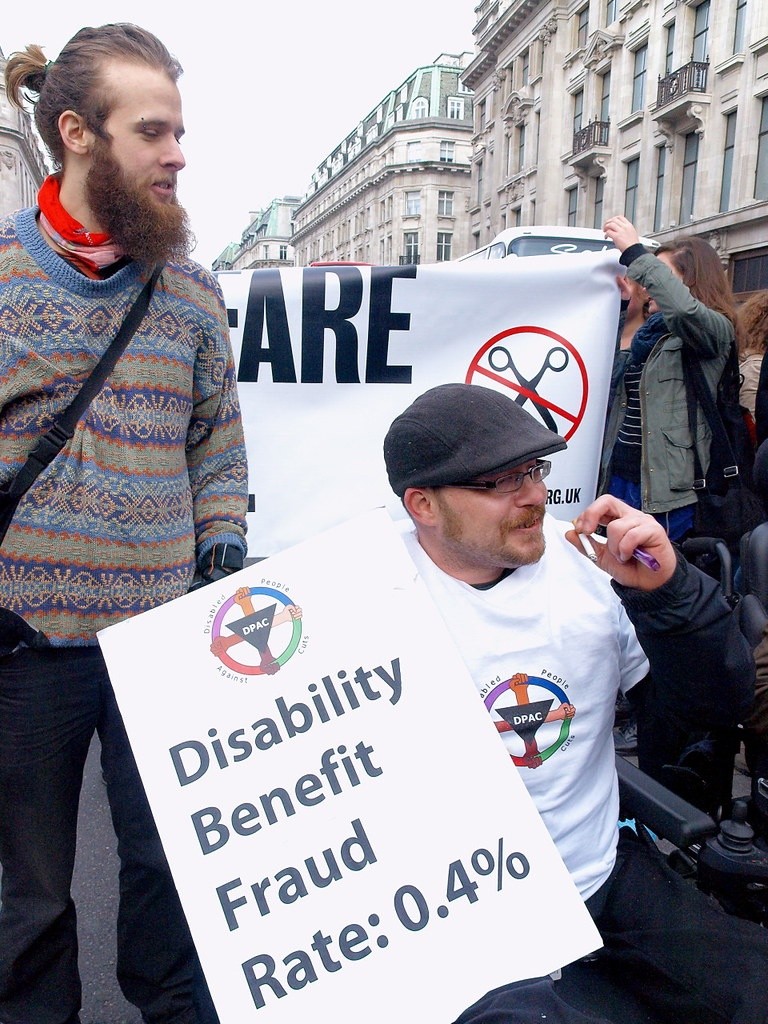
383, 384, 567, 496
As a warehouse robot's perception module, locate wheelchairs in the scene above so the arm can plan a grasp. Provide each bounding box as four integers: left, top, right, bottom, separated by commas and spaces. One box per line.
608, 741, 768, 927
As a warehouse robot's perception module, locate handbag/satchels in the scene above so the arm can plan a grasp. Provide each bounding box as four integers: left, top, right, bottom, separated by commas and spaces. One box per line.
681, 310, 768, 552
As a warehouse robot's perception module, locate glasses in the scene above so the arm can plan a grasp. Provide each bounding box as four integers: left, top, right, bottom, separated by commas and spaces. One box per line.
443, 459, 551, 493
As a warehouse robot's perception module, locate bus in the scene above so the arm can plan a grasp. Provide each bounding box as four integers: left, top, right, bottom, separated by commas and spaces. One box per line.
445, 224, 635, 263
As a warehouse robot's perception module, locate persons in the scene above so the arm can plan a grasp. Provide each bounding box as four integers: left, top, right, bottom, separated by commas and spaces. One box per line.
0, 23, 249, 1024
385, 380, 768, 1024
603, 213, 768, 795
